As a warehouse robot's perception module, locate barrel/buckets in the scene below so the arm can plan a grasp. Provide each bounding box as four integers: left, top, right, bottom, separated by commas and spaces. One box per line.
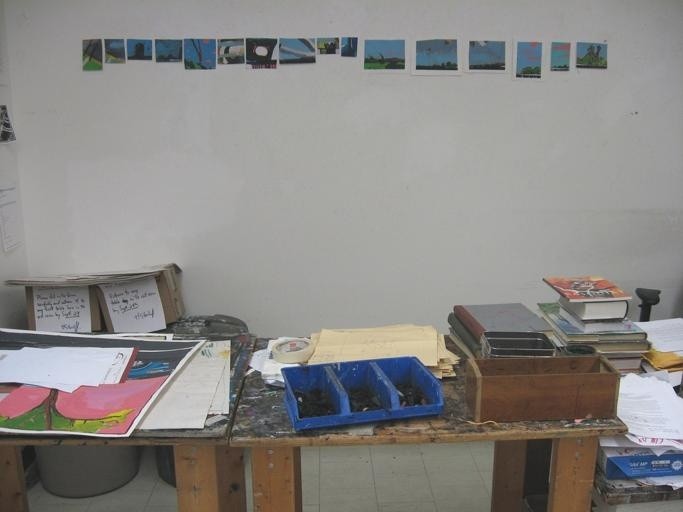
33, 444, 141, 500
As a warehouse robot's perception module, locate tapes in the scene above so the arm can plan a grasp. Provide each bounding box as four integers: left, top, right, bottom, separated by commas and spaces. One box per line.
271, 337, 314, 364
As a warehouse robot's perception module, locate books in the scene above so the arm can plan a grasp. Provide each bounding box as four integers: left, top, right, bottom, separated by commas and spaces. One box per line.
447, 274, 681, 377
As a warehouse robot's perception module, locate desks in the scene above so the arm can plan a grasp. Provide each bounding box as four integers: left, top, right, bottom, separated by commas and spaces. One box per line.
230, 337, 628, 512
592, 463, 683, 512
0, 328, 258, 512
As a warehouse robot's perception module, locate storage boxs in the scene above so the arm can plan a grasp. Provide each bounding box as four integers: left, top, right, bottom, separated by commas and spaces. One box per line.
25, 285, 106, 333
95, 270, 185, 333
